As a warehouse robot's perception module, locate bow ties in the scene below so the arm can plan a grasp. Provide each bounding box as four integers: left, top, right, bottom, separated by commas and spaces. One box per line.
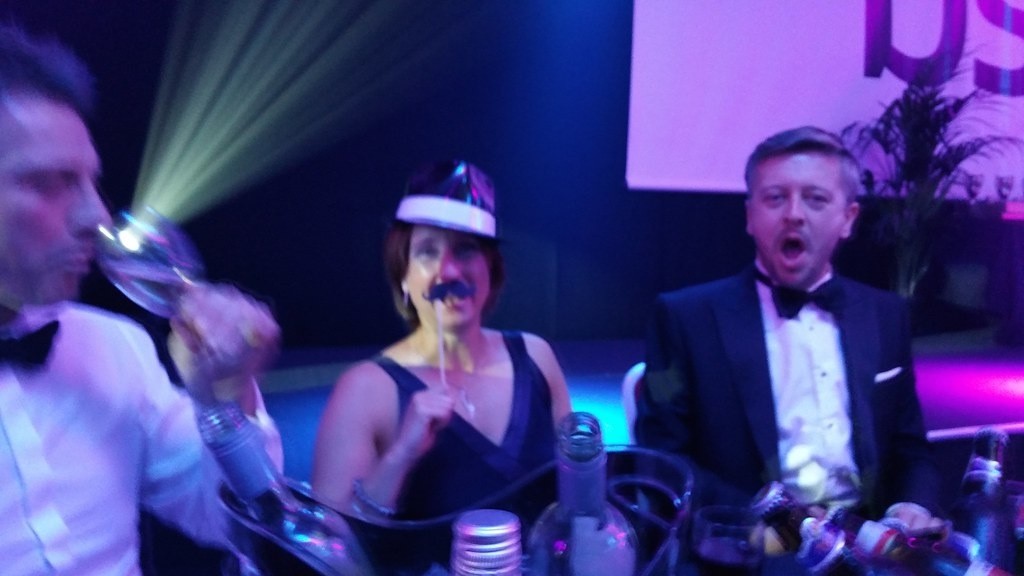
751, 264, 846, 318
0, 317, 61, 366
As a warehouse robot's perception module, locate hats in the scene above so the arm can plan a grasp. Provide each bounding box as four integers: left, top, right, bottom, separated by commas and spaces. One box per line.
393, 160, 498, 240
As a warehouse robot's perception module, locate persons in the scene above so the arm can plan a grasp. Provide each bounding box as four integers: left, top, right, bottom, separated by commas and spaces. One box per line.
310, 159, 573, 576
0, 25, 284, 576
631, 126, 950, 576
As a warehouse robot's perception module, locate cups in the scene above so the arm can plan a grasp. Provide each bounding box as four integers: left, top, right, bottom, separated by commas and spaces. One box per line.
995, 176, 1014, 202
963, 174, 982, 204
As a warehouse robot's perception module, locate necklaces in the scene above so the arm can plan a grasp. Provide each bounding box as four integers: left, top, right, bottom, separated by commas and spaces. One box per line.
422, 335, 491, 419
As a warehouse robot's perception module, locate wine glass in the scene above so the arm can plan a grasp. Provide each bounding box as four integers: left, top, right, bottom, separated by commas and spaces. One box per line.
89, 202, 263, 392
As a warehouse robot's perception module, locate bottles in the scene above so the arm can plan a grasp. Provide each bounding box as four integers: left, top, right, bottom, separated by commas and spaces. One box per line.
198, 403, 374, 576
450, 508, 522, 576
524, 412, 638, 576
754, 428, 1024, 576
